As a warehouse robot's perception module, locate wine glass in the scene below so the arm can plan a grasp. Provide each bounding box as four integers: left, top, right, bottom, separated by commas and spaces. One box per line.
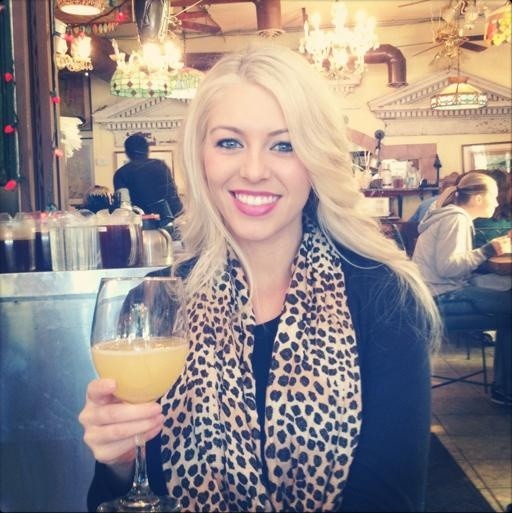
91, 277, 191, 513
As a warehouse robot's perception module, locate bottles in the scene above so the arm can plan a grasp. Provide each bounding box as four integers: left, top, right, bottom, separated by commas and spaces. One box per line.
0, 188, 173, 273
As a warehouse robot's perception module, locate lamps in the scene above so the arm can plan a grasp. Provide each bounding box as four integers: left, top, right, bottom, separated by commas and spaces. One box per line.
428, 0, 511, 74
430, 42, 488, 110
297, 0, 378, 97
59, 116, 84, 160
109, 7, 205, 100
52, 23, 95, 74
54, 0, 108, 16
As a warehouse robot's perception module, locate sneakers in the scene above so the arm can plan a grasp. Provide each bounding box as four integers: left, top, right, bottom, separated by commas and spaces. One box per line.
491, 390, 512, 406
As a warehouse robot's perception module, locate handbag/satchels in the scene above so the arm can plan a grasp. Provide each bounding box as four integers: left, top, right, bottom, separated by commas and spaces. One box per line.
142, 198, 175, 227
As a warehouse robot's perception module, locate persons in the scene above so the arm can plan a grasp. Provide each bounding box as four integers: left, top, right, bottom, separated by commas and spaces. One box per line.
471, 167, 512, 276
404, 170, 460, 225
76, 42, 446, 513
411, 172, 512, 408
110, 132, 187, 240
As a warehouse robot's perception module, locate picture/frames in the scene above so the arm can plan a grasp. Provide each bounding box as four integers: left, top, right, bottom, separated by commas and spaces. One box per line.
461, 141, 512, 176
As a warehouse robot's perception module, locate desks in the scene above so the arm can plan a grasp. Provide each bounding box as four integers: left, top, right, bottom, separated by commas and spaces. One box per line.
0, 181, 512, 513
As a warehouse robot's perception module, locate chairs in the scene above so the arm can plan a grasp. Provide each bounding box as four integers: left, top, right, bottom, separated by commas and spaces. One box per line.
0, 181, 512, 513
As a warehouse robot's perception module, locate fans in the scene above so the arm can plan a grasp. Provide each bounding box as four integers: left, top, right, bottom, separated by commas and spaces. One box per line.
412, 34, 488, 53
